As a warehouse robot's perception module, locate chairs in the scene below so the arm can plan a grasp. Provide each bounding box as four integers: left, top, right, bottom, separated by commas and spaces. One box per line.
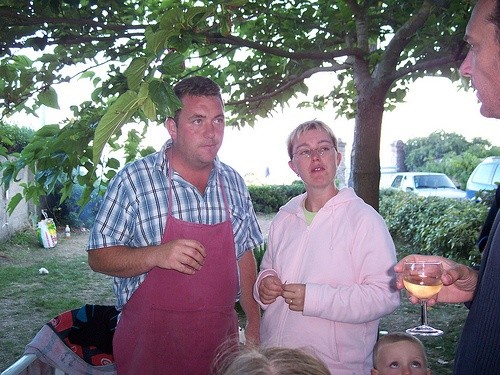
0, 305, 120, 375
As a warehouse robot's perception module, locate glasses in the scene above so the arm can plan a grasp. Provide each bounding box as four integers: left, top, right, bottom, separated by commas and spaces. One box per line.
292, 144, 335, 159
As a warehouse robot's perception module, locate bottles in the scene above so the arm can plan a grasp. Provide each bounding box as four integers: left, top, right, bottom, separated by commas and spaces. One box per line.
65, 224, 70, 240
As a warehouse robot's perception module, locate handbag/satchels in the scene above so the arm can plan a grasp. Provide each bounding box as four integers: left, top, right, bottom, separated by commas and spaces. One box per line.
39, 218, 57, 248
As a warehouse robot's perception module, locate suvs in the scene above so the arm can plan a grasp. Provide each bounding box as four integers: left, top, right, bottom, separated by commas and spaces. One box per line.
390, 172, 468, 199
466, 156, 500, 200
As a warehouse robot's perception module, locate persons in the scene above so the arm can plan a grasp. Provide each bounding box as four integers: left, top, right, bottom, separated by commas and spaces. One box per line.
394, 0, 500, 375
85, 76, 264, 375
252, 118, 400, 375
371, 332, 430, 375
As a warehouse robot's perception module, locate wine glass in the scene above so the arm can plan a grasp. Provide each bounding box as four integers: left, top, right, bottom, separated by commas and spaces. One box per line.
403, 261, 445, 336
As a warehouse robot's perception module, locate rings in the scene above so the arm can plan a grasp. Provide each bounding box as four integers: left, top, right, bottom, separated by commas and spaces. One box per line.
291, 299, 293, 305
